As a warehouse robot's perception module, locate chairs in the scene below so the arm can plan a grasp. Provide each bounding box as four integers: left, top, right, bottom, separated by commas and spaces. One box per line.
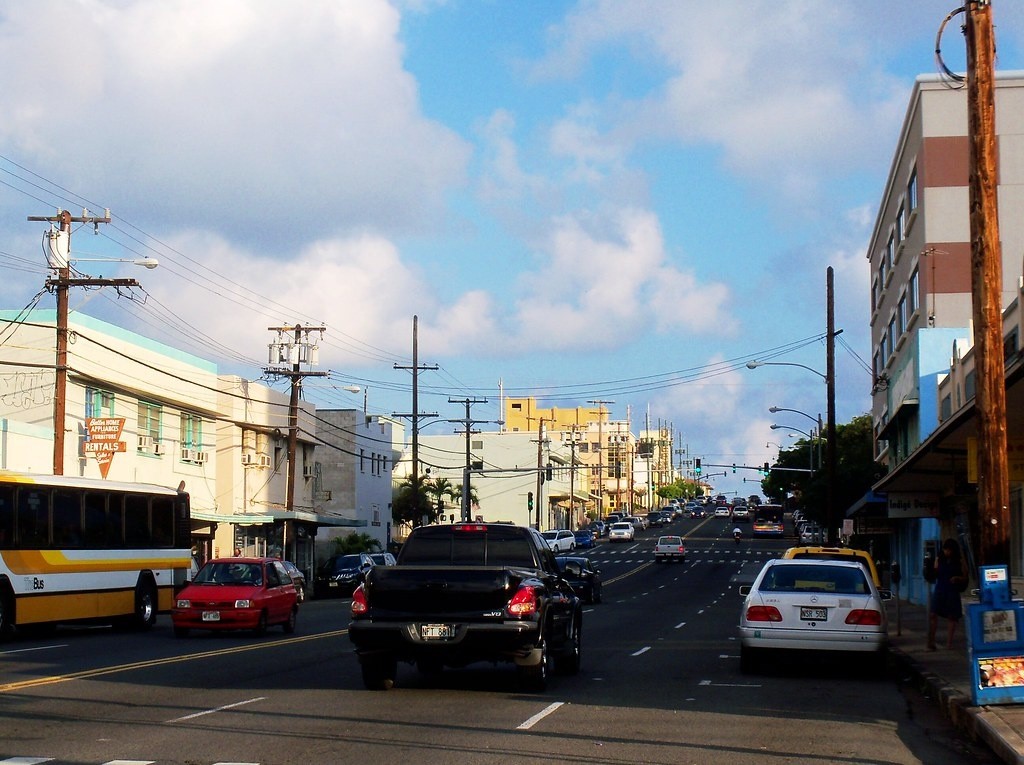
774, 571, 796, 589
835, 574, 855, 593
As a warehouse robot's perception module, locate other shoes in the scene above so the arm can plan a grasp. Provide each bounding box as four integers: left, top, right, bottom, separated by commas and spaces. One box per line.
947, 640, 954, 650
927, 637, 937, 649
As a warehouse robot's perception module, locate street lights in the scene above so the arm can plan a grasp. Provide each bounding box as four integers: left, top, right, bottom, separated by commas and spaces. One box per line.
770, 424, 813, 479
746, 360, 835, 518
287, 383, 360, 510
769, 405, 822, 469
53, 257, 159, 475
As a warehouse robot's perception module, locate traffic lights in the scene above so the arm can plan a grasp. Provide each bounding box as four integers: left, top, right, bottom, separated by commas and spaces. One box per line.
696, 459, 701, 473
764, 463, 769, 476
528, 492, 533, 511
733, 463, 736, 473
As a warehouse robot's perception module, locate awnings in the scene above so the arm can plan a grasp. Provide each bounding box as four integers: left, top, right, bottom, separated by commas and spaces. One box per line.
258, 511, 367, 527
870, 347, 1024, 494
189, 511, 274, 531
846, 493, 887, 518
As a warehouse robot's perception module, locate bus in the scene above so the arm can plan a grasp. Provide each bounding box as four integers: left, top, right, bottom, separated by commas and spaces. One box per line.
0, 467, 193, 631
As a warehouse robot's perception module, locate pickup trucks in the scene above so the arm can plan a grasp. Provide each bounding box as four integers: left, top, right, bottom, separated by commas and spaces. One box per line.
346, 521, 583, 690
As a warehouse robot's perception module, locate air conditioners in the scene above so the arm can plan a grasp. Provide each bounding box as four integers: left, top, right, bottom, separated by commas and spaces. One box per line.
305, 465, 316, 476
196, 452, 209, 462
153, 444, 161, 455
181, 448, 194, 462
261, 456, 272, 466
137, 436, 154, 449
241, 453, 252, 464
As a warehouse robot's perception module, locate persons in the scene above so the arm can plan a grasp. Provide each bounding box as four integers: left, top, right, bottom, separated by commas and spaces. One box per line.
929, 538, 970, 649
732, 525, 742, 539
231, 548, 243, 557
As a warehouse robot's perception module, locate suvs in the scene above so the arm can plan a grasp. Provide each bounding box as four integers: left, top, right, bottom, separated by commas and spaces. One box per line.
777, 546, 883, 591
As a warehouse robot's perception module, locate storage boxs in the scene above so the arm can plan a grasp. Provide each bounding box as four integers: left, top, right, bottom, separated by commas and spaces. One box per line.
979, 563, 1011, 607
966, 647, 1024, 703
961, 594, 1024, 652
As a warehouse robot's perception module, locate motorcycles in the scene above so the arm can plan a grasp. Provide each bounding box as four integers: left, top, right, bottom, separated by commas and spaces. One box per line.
735, 532, 740, 544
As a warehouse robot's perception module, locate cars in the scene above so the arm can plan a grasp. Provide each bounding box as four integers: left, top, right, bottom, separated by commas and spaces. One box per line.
554, 557, 603, 602
650, 495, 821, 544
738, 558, 892, 663
654, 536, 685, 563
172, 551, 397, 637
541, 512, 649, 558
